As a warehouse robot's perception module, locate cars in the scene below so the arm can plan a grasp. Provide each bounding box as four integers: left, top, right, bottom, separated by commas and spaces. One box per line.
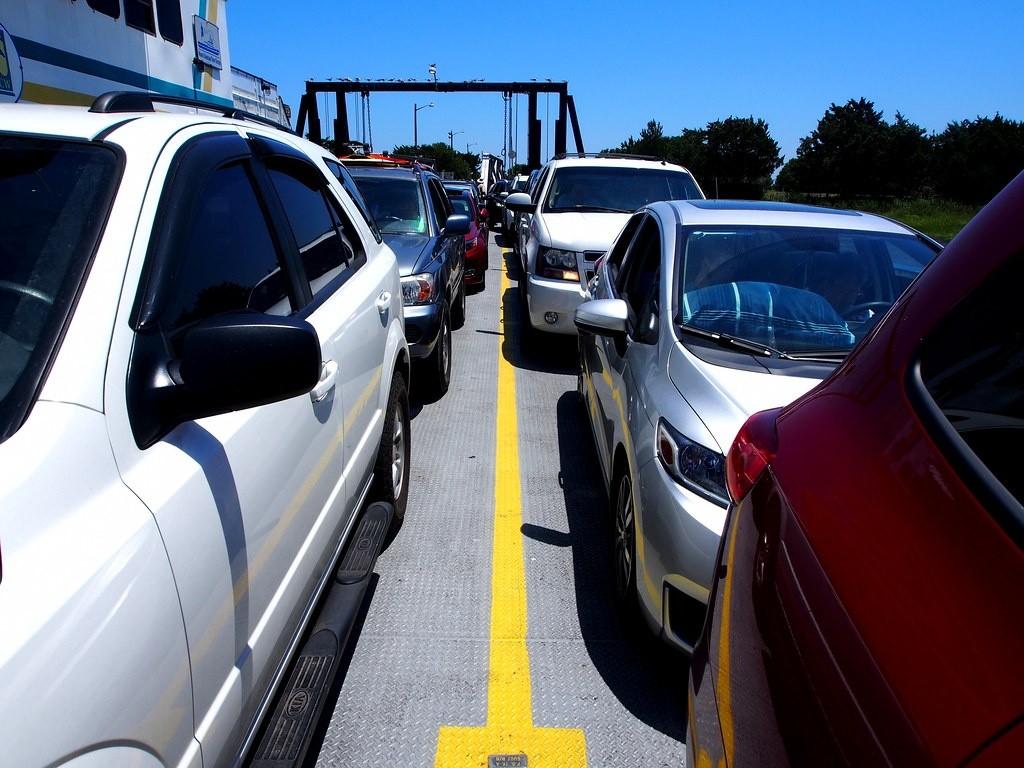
572, 199, 947, 656
486, 169, 543, 282
440, 178, 490, 295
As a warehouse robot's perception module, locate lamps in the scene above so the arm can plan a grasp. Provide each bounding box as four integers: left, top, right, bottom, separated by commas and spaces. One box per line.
429, 64, 437, 83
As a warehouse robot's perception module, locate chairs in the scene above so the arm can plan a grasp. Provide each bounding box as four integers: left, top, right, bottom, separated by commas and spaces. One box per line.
807, 243, 854, 307
453, 202, 465, 215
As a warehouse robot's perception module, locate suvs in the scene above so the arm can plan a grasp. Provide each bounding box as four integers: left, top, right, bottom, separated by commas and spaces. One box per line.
503, 156, 706, 348
0, 92, 413, 768
684, 164, 1024, 767
340, 161, 466, 399
